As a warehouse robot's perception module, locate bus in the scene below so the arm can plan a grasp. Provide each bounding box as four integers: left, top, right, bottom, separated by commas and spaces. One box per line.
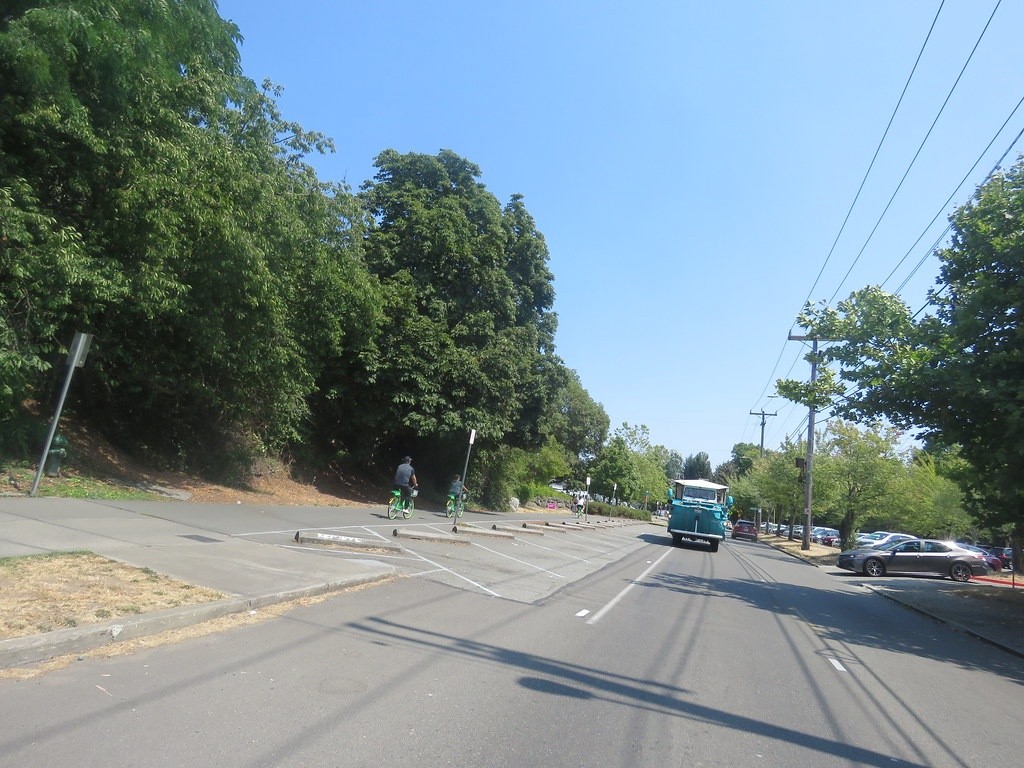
666, 479, 734, 553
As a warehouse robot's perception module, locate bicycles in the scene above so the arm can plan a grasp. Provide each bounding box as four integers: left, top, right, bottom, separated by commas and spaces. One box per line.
387, 485, 420, 520
445, 490, 470, 518
576, 506, 585, 520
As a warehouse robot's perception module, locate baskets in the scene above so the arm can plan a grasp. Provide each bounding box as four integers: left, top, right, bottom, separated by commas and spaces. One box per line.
462, 494, 466, 500
411, 489, 418, 497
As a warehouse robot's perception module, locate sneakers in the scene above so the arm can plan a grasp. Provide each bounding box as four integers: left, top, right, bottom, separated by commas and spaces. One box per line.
403, 509, 410, 513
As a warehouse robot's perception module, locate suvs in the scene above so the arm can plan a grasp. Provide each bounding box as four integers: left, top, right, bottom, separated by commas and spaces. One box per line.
731, 519, 759, 542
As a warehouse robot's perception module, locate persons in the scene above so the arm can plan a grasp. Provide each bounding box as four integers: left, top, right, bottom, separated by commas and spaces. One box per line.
572, 494, 587, 516
448, 474, 469, 513
393, 456, 418, 513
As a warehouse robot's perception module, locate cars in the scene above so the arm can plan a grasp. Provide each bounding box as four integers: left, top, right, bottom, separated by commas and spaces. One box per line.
927, 542, 1002, 573
985, 546, 1024, 570
760, 520, 922, 548
836, 538, 989, 582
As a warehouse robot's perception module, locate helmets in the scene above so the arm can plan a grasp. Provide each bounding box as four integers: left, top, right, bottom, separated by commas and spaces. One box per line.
454, 474, 460, 479
405, 456, 412, 461
